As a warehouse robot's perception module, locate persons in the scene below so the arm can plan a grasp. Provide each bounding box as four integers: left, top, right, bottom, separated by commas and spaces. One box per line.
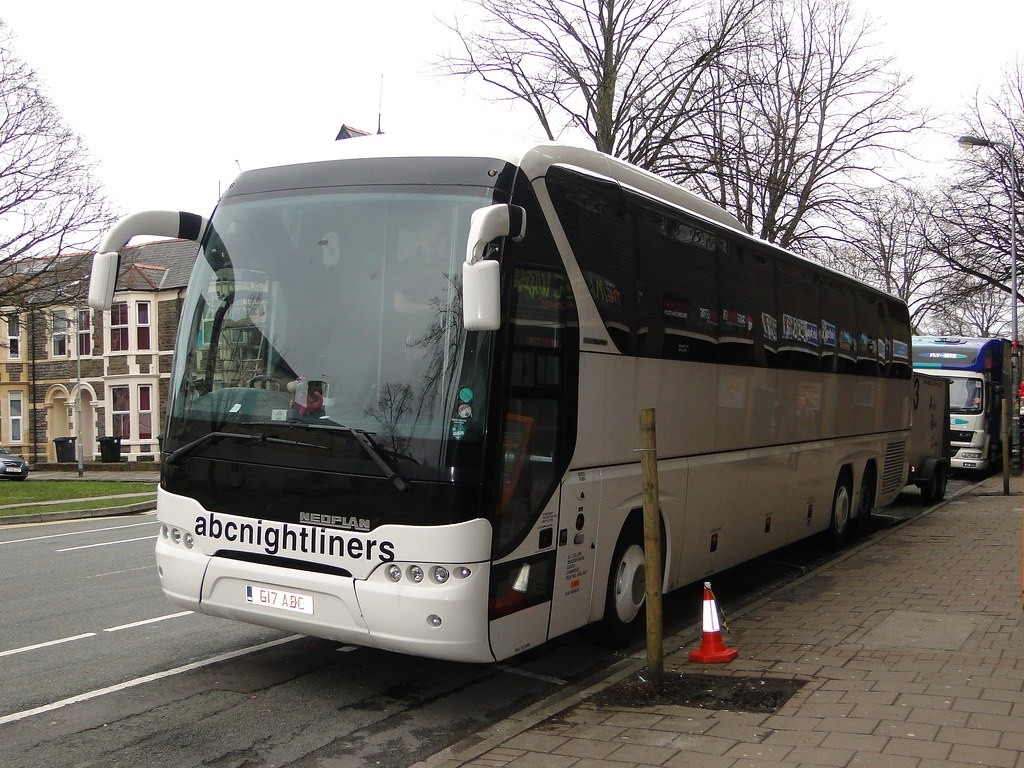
389, 226, 449, 418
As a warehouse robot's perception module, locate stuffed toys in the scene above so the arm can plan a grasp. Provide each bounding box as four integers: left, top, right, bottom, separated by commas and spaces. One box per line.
286, 376, 323, 417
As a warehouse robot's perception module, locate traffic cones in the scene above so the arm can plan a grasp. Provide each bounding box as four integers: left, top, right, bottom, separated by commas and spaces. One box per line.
688, 581, 738, 663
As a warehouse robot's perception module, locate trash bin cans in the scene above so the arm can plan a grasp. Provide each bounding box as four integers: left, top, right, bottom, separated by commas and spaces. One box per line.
156, 434, 163, 458
96, 436, 123, 463
53, 437, 78, 463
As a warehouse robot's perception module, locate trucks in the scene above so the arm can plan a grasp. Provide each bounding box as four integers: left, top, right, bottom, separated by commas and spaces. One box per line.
911, 336, 1024, 475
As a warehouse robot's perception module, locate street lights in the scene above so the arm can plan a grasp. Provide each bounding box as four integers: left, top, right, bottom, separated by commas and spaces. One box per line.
958, 136, 1022, 477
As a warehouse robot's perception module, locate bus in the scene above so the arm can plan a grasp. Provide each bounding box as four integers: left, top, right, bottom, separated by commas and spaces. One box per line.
85, 143, 914, 668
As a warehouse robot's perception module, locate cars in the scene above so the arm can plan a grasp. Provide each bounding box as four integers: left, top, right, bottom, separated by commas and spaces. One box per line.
0, 445, 30, 481
